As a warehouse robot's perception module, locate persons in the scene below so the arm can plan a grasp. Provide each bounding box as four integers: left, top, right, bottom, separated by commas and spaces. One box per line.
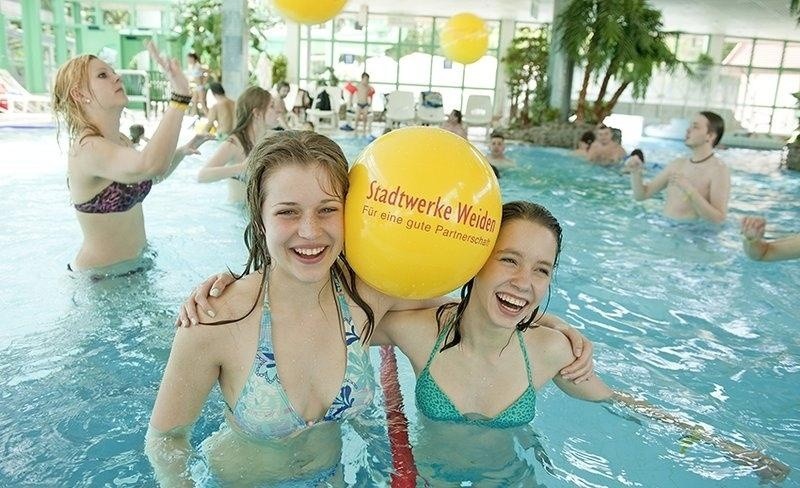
738, 217, 800, 263
586, 126, 626, 163
622, 150, 643, 172
274, 81, 298, 132
52, 39, 218, 284
625, 111, 731, 222
440, 110, 467, 140
128, 125, 150, 151
203, 82, 235, 141
302, 121, 314, 132
485, 134, 514, 166
145, 129, 595, 488
186, 52, 216, 134
290, 106, 300, 127
172, 200, 616, 488
198, 85, 283, 204
569, 131, 593, 157
350, 72, 372, 135
129, 39, 149, 118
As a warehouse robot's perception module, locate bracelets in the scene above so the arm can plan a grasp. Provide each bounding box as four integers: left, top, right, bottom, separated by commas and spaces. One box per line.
168, 92, 191, 110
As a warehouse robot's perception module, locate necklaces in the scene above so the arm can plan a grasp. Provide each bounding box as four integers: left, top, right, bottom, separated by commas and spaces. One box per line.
689, 152, 715, 163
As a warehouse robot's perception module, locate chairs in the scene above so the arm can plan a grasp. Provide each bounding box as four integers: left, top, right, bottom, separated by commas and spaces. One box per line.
384, 91, 415, 129
120, 70, 151, 119
462, 95, 492, 140
306, 87, 341, 131
416, 91, 442, 126
